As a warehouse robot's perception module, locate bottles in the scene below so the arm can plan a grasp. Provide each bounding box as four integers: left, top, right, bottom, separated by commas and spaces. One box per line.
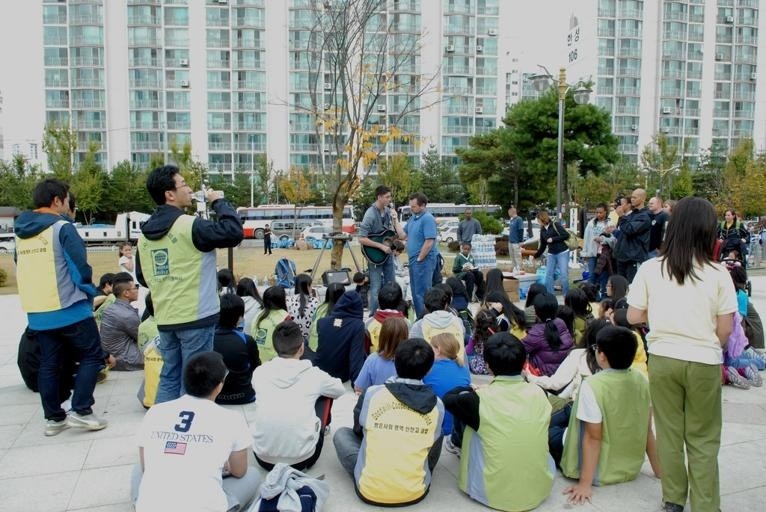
470, 232, 497, 269
406, 299, 415, 322
191, 190, 226, 203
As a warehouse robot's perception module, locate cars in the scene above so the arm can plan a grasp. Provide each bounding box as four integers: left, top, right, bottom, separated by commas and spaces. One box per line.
742, 220, 766, 240
436, 208, 564, 247
300, 225, 353, 242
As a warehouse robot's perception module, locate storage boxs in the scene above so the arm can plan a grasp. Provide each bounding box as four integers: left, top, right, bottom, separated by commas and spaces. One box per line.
502, 275, 520, 302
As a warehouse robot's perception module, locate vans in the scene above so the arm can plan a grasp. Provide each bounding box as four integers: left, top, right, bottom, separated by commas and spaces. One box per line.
271, 220, 324, 241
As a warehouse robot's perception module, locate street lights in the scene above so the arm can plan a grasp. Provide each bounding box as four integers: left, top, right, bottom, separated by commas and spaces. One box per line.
526, 70, 592, 223
642, 164, 681, 203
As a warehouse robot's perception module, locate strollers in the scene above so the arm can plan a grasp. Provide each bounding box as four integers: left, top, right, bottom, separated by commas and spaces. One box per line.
716, 238, 753, 297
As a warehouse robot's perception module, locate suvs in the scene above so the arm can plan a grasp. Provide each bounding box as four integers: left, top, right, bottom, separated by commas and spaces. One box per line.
0, 233, 16, 253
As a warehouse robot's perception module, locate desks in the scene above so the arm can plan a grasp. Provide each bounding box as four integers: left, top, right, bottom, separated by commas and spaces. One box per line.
514, 272, 542, 297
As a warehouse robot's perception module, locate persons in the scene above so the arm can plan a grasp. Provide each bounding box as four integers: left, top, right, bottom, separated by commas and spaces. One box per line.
135, 322, 346, 510
316, 183, 765, 512
14, 166, 320, 436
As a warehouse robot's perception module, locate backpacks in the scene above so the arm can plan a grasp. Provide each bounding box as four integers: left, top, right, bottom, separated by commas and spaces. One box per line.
274, 256, 297, 289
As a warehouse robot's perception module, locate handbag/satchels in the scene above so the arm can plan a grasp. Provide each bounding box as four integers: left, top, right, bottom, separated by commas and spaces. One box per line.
565, 229, 579, 251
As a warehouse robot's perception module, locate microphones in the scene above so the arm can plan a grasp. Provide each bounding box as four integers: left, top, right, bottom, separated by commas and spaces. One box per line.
389, 203, 394, 208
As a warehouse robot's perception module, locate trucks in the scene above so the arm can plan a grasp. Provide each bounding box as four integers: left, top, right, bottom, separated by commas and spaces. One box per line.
74, 210, 151, 246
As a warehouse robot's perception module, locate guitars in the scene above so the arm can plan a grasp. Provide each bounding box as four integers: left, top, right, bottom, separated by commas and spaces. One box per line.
361, 230, 400, 265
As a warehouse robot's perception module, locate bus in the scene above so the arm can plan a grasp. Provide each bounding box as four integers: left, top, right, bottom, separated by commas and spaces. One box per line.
236, 203, 356, 238
396, 204, 502, 231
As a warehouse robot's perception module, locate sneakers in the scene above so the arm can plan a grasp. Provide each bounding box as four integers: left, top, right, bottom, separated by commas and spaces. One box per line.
43, 418, 65, 437
727, 362, 764, 389
65, 409, 110, 431
745, 345, 760, 359
